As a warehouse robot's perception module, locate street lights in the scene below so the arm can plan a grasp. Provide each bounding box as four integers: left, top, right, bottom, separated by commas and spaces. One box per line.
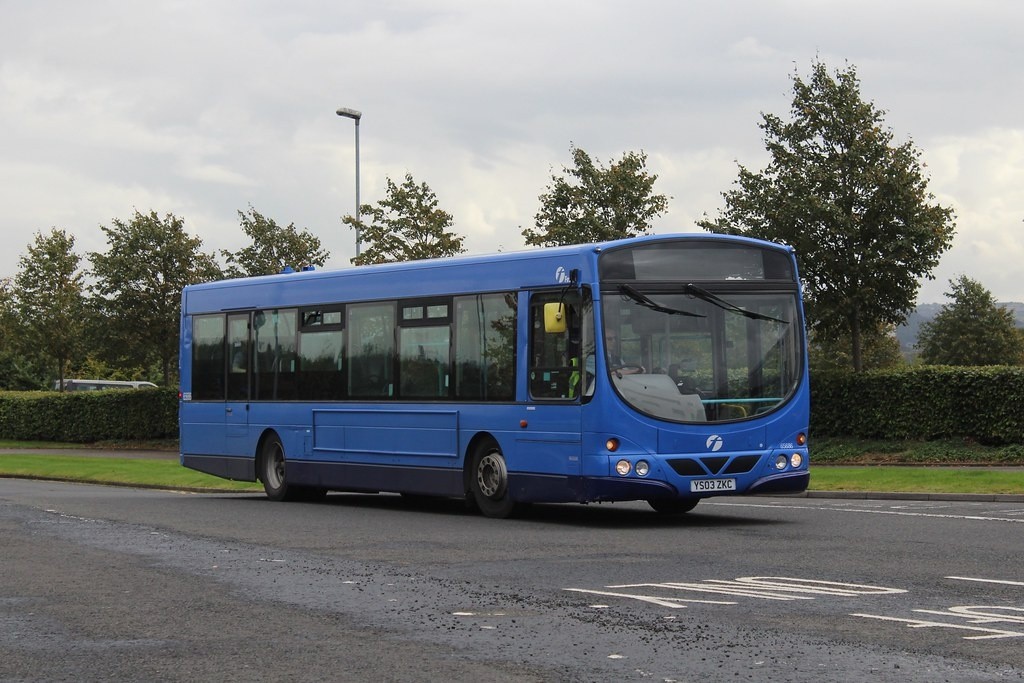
335, 106, 362, 265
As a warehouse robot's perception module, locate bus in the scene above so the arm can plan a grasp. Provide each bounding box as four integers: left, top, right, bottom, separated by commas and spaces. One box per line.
54, 379, 158, 392
175, 232, 813, 517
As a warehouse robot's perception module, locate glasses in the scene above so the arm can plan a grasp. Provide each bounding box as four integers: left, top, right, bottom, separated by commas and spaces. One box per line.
606, 336, 617, 341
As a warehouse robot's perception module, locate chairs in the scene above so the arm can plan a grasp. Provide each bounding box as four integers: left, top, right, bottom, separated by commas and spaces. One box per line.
566, 357, 581, 397
211, 344, 451, 399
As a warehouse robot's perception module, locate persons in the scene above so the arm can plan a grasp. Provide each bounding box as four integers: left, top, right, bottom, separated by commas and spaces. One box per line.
584, 329, 644, 394
485, 357, 506, 388
